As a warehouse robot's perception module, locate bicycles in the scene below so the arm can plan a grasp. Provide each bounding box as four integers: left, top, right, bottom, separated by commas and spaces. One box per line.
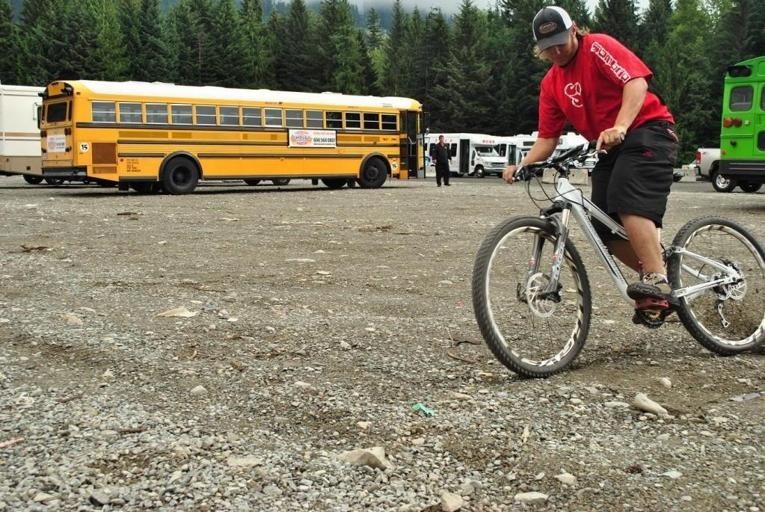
470, 136, 764, 378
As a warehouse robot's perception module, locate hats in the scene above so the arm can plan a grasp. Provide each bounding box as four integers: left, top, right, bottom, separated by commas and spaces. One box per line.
531, 5, 573, 54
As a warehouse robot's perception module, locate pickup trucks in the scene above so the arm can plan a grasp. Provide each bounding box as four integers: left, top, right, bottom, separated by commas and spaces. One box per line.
696, 147, 762, 192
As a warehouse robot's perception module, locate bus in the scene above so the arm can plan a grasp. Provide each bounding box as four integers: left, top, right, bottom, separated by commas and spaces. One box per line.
718, 55, 765, 181
37, 78, 431, 195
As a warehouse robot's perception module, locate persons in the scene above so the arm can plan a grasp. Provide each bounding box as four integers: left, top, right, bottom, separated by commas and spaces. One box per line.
503, 6, 681, 324
433, 135, 453, 186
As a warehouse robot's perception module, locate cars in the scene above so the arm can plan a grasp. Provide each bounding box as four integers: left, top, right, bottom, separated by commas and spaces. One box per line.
672, 159, 696, 183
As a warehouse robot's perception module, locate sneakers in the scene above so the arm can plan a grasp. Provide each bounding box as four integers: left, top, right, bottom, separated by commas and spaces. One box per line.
437, 183, 441, 187
632, 310, 674, 325
444, 183, 452, 186
626, 272, 682, 309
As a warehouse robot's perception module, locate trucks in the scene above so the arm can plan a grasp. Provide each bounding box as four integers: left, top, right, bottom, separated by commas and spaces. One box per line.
423, 131, 599, 179
0, 85, 62, 187
